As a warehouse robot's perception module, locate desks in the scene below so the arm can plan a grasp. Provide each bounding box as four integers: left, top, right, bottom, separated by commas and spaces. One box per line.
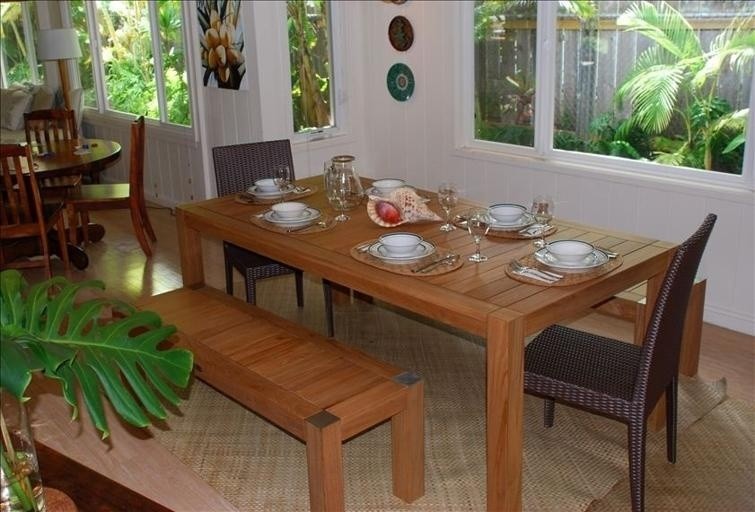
0, 140, 123, 240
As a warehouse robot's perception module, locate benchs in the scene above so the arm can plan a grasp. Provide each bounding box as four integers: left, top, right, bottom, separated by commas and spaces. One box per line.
112, 283, 424, 512
588, 274, 707, 376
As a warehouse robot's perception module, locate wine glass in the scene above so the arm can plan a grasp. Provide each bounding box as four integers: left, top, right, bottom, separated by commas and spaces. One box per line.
463, 207, 489, 263
321, 178, 352, 222
436, 182, 459, 233
530, 194, 555, 248
271, 178, 289, 204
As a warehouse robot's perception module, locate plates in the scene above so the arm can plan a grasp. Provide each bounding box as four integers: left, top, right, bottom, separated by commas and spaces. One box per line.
372, 179, 406, 195
386, 63, 414, 102
271, 202, 306, 218
265, 208, 323, 225
247, 183, 295, 200
365, 186, 417, 197
546, 239, 594, 262
489, 204, 527, 223
388, 16, 414, 51
479, 213, 535, 230
368, 241, 436, 260
535, 248, 608, 271
255, 177, 283, 192
378, 231, 424, 256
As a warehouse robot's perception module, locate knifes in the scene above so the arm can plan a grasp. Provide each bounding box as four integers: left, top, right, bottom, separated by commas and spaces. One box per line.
287, 221, 330, 231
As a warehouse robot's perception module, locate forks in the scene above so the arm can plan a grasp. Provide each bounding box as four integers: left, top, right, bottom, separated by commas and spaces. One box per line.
412, 253, 460, 273
505, 259, 553, 272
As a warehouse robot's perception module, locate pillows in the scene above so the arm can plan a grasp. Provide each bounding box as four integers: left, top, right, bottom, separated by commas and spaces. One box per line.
56, 87, 84, 133
9, 82, 29, 94
1, 88, 33, 132
32, 85, 55, 130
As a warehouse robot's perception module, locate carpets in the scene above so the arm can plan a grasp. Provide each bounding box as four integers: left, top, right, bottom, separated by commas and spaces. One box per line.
103, 270, 755, 512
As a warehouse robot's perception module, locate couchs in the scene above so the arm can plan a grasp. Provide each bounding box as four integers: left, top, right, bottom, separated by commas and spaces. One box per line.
0, 87, 85, 145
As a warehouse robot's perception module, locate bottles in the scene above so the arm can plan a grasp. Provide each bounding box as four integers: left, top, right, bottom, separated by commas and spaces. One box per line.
327, 178, 364, 211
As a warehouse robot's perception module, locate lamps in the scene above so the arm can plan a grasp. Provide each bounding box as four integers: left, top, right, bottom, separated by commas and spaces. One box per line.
38, 29, 85, 137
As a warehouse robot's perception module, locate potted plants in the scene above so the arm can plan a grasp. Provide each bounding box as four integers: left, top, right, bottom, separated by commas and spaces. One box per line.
0, 270, 194, 512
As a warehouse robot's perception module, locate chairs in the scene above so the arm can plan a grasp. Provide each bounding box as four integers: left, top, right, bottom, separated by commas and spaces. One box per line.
0, 144, 73, 297
524, 213, 717, 512
13, 109, 90, 247
212, 138, 340, 339
70, 116, 156, 258
